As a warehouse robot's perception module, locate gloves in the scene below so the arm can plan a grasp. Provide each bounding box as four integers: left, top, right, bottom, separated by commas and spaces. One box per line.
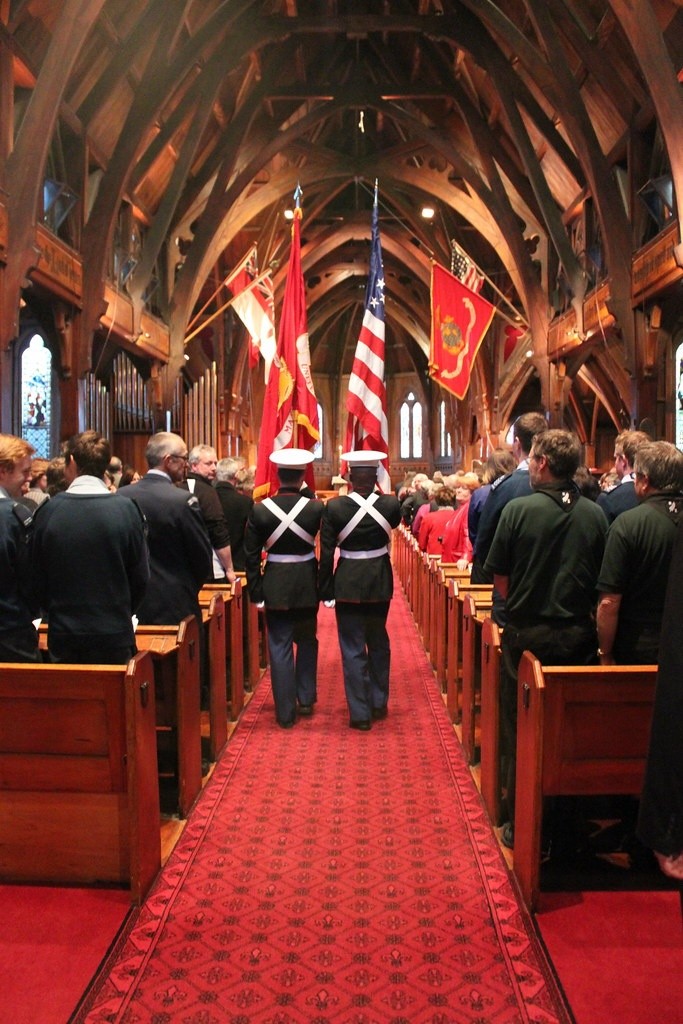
323, 599, 336, 608
255, 600, 265, 609
299, 480, 307, 492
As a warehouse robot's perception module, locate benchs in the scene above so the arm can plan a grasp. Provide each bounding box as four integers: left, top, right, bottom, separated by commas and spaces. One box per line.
389, 486, 683, 910
0, 487, 269, 896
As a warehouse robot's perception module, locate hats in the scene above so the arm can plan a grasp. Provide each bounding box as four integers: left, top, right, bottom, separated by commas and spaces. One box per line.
340, 450, 388, 467
269, 448, 315, 469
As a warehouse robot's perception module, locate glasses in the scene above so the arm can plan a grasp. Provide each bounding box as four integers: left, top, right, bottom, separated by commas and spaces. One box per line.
629, 472, 639, 479
163, 454, 189, 462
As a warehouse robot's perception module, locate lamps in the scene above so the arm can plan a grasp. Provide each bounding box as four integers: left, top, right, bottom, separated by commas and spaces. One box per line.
422, 201, 436, 219
281, 202, 294, 222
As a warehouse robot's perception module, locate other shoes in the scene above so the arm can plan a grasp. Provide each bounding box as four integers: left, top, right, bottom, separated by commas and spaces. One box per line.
372, 706, 387, 717
276, 717, 295, 726
503, 823, 515, 848
299, 704, 312, 713
350, 720, 369, 729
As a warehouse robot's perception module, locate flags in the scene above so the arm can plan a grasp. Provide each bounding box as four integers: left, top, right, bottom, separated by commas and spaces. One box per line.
340, 204, 392, 495
451, 245, 485, 294
232, 269, 276, 385
226, 249, 259, 369
253, 208, 319, 501
426, 260, 496, 403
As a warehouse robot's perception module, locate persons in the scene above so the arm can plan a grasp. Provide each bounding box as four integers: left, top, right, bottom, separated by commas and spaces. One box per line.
395, 411, 683, 908
0, 431, 257, 778
243, 448, 324, 729
320, 450, 402, 729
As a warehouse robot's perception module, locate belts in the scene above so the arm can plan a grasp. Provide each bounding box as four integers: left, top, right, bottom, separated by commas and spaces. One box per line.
341, 545, 388, 559
267, 551, 314, 562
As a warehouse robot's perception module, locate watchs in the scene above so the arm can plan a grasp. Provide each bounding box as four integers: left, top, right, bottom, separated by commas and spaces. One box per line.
596, 648, 610, 656
